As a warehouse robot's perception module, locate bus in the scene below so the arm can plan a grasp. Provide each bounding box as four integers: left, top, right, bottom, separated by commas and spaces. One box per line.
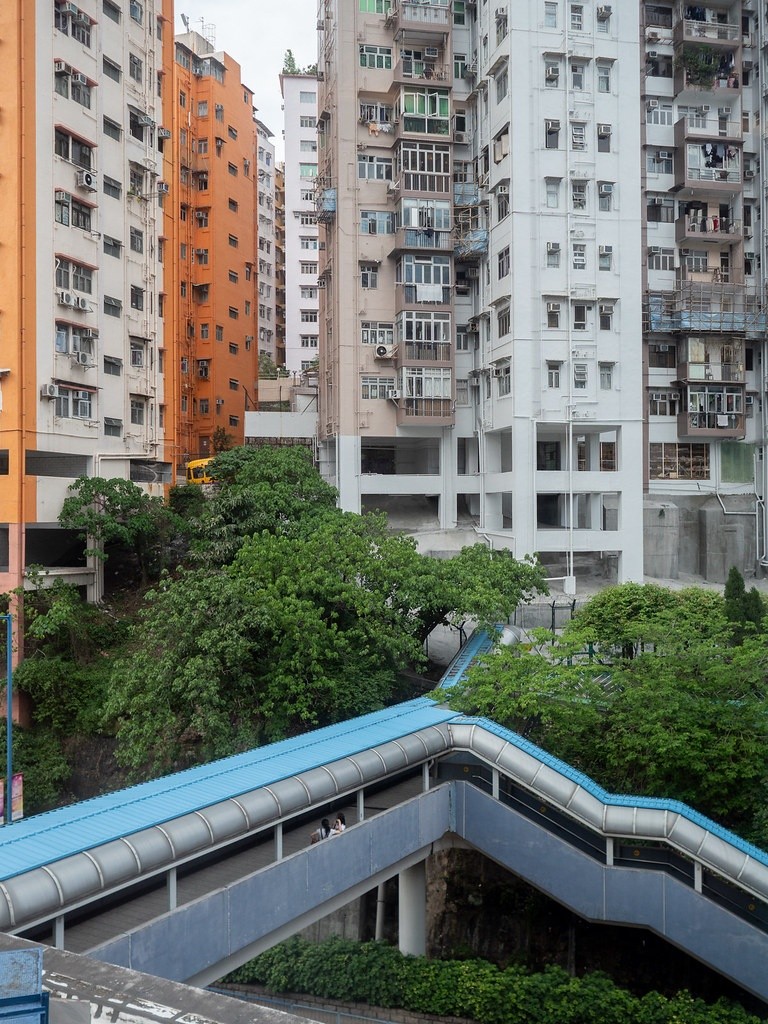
184, 456, 222, 487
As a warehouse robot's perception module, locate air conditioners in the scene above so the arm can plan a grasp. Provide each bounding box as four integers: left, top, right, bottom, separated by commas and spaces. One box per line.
196, 212, 203, 217
55, 62, 72, 75
244, 160, 251, 166
466, 267, 478, 278
652, 393, 661, 400
158, 183, 168, 193
200, 361, 206, 366
685, 28, 694, 36
547, 303, 562, 312
647, 100, 659, 108
467, 0, 475, 6
600, 245, 613, 255
601, 305, 614, 315
745, 251, 755, 260
746, 396, 753, 404
387, 391, 400, 399
85, 329, 98, 339
317, 20, 324, 30
76, 391, 88, 399
599, 5, 611, 18
679, 248, 690, 254
217, 139, 223, 146
78, 171, 97, 191
743, 61, 753, 69
649, 32, 659, 39
469, 378, 479, 386
744, 226, 752, 236
62, 3, 78, 16
548, 121, 560, 131
744, 170, 755, 178
649, 51, 657, 57
216, 400, 224, 405
719, 107, 731, 114
77, 351, 90, 365
294, 212, 299, 216
660, 344, 668, 351
706, 369, 714, 381
668, 393, 680, 400
653, 197, 664, 205
56, 192, 71, 202
698, 106, 711, 114
599, 125, 612, 137
138, 117, 152, 126
496, 186, 508, 197
59, 292, 74, 306
468, 324, 476, 333
495, 7, 505, 18
375, 345, 392, 358
386, 9, 393, 22
660, 151, 668, 159
76, 296, 89, 310
195, 68, 202, 76
158, 129, 171, 139
387, 182, 395, 192
73, 73, 87, 86
547, 242, 560, 252
547, 67, 559, 79
425, 47, 438, 58
741, 38, 751, 45
454, 131, 469, 143
465, 65, 477, 72
42, 384, 59, 397
75, 13, 91, 26
648, 246, 659, 254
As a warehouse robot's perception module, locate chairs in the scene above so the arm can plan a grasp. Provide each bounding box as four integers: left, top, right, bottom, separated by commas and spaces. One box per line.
718, 171, 727, 181
